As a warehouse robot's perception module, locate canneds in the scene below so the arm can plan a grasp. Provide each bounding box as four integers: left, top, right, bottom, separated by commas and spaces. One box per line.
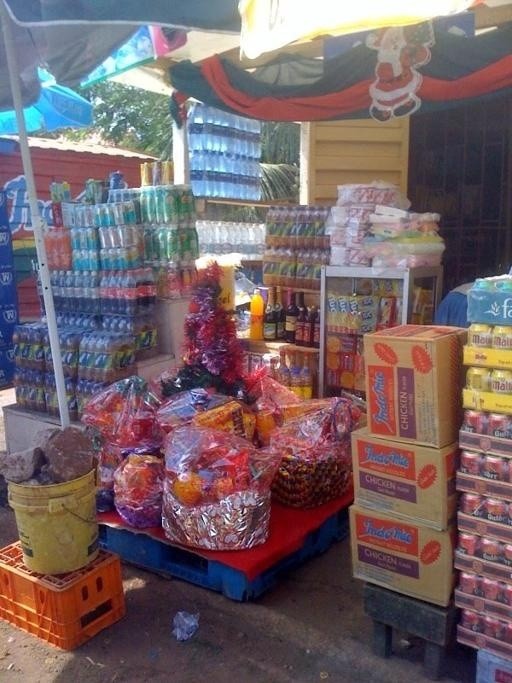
459, 274, 512, 648
67, 202, 142, 270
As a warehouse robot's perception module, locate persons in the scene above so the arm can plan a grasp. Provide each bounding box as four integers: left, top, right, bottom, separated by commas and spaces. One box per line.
433, 263, 484, 328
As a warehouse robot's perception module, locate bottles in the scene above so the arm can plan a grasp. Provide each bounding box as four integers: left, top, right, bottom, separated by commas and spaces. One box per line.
249, 285, 321, 349
138, 184, 199, 299
11, 188, 159, 423
194, 219, 264, 261
262, 205, 330, 290
277, 351, 314, 400
187, 102, 262, 202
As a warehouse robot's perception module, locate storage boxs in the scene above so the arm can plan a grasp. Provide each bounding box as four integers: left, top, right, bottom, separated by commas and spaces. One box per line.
363, 322, 468, 448
349, 505, 460, 608
476, 650, 512, 683
351, 426, 462, 531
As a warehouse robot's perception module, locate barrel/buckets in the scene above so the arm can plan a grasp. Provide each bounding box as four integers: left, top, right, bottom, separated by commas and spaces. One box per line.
6, 469, 99, 574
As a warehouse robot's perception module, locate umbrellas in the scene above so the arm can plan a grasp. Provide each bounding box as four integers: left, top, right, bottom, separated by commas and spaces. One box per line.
0, 0, 242, 430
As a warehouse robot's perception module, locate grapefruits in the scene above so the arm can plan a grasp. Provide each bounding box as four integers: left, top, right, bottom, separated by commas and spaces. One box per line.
175, 471, 205, 504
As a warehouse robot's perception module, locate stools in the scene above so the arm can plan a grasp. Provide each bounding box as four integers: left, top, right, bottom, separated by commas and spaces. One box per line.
361, 583, 461, 681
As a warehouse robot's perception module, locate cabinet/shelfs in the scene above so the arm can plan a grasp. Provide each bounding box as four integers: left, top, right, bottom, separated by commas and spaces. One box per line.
3, 195, 444, 455
